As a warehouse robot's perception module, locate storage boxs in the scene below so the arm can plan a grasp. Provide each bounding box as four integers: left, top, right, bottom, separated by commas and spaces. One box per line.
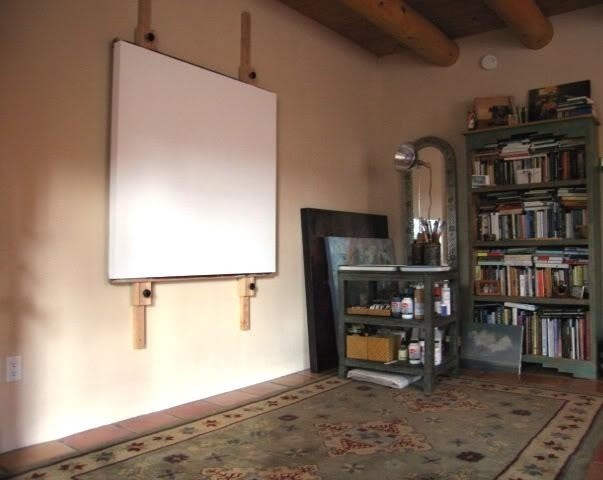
345, 334, 367, 361
366, 334, 401, 363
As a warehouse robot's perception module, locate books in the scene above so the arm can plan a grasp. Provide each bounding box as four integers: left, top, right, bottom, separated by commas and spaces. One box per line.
471, 95, 592, 362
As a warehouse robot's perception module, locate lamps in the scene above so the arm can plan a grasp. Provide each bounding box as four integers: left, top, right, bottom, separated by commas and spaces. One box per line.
391, 139, 440, 232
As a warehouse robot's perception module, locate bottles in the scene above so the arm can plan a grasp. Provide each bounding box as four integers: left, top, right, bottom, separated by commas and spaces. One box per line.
561, 148, 570, 180
397, 326, 441, 367
391, 281, 451, 320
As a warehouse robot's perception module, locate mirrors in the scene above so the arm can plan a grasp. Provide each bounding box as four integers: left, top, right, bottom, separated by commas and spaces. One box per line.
400, 135, 459, 268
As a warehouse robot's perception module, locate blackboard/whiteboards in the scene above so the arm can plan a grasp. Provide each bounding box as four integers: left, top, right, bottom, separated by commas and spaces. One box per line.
106, 37, 277, 283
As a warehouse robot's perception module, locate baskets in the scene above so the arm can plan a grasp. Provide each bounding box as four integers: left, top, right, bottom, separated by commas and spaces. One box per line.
347, 307, 392, 317
346, 333, 400, 363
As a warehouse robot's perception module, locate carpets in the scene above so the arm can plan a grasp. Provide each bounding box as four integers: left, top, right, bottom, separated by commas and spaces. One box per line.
2, 366, 603, 479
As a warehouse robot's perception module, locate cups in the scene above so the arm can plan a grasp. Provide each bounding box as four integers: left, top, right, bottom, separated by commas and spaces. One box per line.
410, 242, 440, 266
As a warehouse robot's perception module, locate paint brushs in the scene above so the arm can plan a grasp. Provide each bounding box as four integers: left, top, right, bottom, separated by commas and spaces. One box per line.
421, 217, 446, 242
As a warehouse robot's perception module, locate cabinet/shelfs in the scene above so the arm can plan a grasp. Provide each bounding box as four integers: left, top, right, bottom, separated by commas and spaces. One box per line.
331, 262, 463, 396
460, 114, 601, 382
